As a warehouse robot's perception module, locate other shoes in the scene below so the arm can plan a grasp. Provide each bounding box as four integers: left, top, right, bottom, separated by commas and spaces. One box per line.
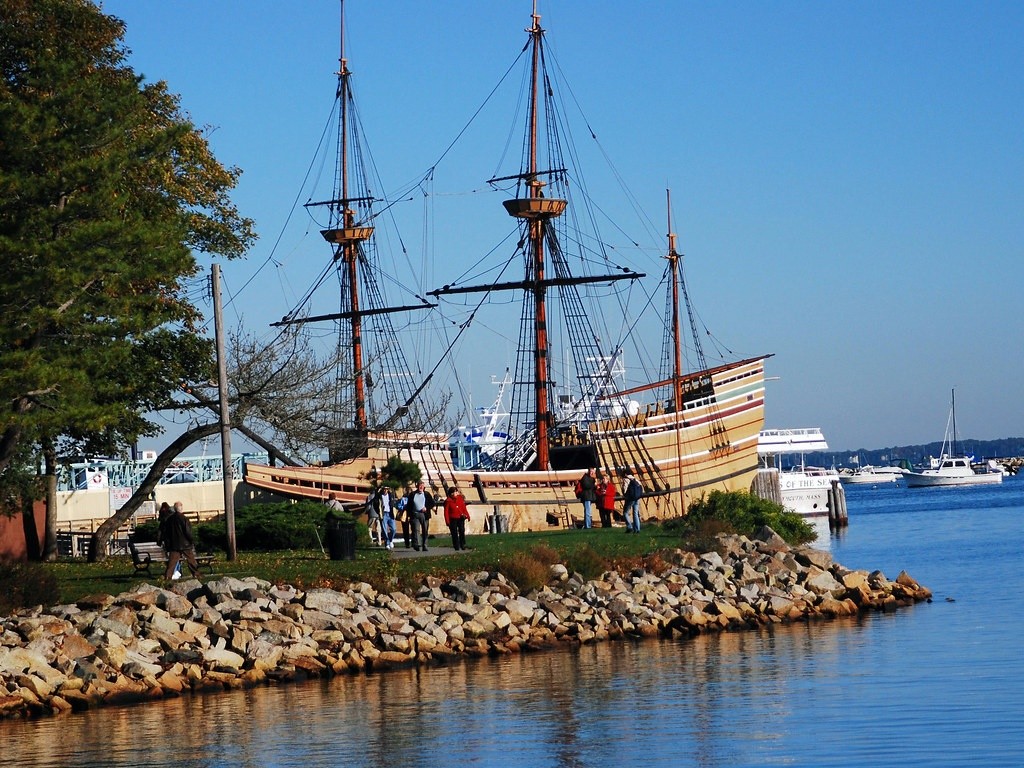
626, 528, 633, 532
415, 546, 420, 551
386, 544, 391, 550
376, 542, 381, 546
422, 544, 429, 551
390, 542, 394, 548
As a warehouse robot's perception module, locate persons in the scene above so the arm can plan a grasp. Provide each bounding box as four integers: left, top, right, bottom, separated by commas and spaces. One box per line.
325, 492, 344, 512
444, 486, 471, 551
574, 467, 600, 529
158, 502, 205, 580
596, 473, 616, 527
365, 487, 382, 546
394, 487, 414, 548
407, 482, 435, 551
621, 470, 644, 533
374, 485, 396, 549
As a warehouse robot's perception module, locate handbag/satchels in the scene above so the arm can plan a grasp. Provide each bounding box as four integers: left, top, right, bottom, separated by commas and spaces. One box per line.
395, 508, 407, 522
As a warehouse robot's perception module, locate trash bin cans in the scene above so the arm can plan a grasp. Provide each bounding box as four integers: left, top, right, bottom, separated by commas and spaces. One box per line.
324, 516, 358, 561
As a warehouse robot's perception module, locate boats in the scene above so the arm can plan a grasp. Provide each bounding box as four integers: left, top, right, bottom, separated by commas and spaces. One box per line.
790, 455, 914, 484
758, 426, 843, 516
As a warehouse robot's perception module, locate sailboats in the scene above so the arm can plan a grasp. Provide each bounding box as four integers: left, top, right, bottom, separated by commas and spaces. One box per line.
899, 387, 1004, 487
243, 1, 776, 537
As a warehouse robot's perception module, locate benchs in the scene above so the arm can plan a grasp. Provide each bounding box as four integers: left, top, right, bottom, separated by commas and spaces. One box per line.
127, 541, 217, 580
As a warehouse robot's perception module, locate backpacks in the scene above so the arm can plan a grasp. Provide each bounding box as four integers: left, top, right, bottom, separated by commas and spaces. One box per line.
575, 480, 583, 499
626, 477, 642, 502
